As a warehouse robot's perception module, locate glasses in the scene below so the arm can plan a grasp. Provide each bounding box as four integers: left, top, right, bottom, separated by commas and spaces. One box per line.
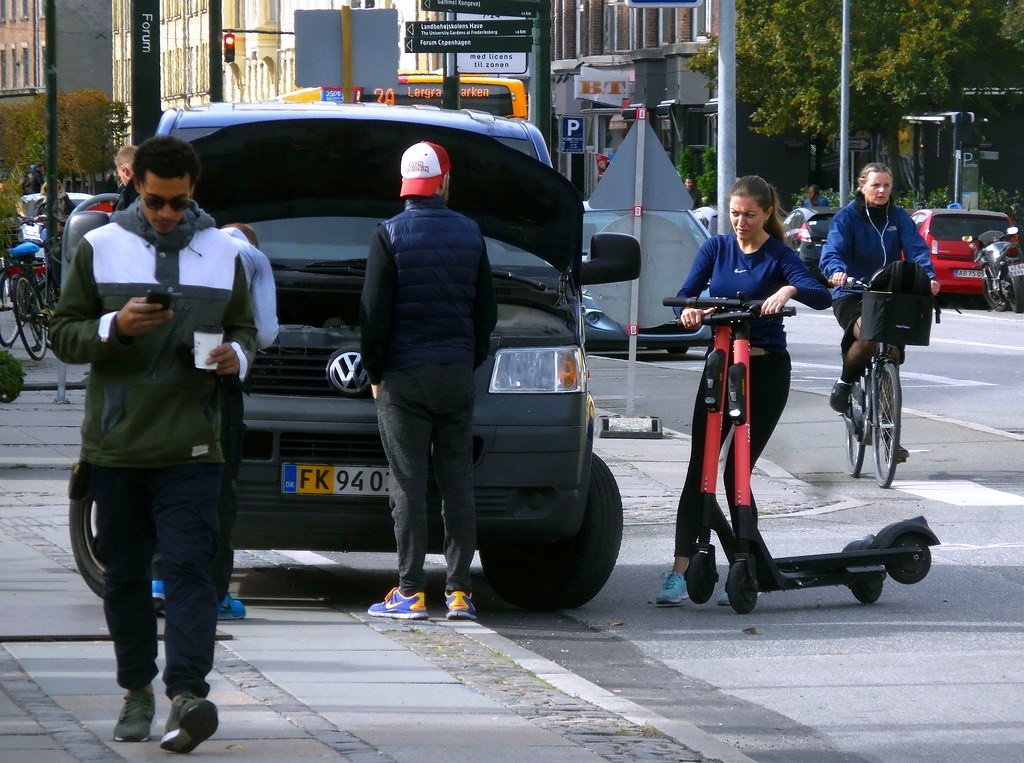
140, 181, 188, 212
114, 170, 123, 180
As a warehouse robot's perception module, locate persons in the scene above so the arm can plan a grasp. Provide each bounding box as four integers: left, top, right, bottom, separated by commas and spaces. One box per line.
111, 143, 138, 212
23, 163, 78, 298
655, 174, 833, 608
48, 136, 256, 754
357, 142, 497, 619
150, 226, 280, 622
801, 184, 829, 207
819, 163, 942, 468
682, 177, 702, 210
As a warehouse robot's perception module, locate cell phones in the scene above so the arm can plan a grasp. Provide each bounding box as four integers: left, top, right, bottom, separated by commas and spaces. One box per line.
145, 289, 173, 313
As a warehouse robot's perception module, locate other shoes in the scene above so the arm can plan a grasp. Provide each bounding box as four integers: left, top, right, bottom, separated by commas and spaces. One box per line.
829, 381, 852, 413
884, 441, 910, 458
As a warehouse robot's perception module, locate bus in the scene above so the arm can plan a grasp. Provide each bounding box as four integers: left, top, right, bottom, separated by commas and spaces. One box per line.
274, 70, 528, 119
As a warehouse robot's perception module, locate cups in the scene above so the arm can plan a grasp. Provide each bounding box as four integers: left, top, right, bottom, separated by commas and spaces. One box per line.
193, 324, 225, 369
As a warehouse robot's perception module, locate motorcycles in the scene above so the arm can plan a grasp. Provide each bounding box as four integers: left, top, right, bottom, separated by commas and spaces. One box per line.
962, 227, 1024, 313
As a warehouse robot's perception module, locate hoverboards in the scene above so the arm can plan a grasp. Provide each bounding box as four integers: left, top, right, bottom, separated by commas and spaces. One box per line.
662, 290, 942, 615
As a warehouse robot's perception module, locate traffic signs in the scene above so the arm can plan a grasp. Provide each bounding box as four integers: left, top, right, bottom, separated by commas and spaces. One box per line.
403, 21, 534, 53
831, 136, 872, 153
420, 0, 540, 18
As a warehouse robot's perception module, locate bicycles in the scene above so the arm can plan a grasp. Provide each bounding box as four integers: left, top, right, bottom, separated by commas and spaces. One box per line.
0, 215, 70, 362
829, 276, 940, 488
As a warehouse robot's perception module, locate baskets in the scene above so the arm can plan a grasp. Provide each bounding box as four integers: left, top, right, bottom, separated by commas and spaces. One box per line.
858, 291, 931, 345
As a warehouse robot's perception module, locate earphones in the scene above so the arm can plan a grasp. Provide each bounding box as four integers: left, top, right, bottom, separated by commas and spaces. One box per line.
861, 187, 865, 194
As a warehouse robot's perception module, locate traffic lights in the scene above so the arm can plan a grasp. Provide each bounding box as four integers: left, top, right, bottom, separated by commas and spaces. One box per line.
224, 33, 236, 63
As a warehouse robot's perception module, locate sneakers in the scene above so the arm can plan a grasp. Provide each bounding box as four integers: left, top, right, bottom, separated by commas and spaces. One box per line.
444, 591, 477, 620
217, 591, 246, 620
657, 570, 689, 604
719, 591, 763, 606
152, 579, 167, 617
367, 587, 429, 620
160, 692, 218, 753
113, 687, 155, 741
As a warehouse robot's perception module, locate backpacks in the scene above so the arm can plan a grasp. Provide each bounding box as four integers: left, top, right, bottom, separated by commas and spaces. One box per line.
872, 258, 933, 344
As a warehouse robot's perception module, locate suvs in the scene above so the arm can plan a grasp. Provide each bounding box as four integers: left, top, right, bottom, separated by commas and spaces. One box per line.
65, 105, 643, 612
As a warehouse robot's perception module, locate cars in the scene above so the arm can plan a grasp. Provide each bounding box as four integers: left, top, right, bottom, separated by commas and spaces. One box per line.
18, 193, 94, 266
901, 208, 1020, 310
579, 201, 718, 355
780, 208, 842, 288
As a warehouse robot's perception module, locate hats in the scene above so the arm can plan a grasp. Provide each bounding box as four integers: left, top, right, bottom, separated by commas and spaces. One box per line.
400, 141, 451, 196
